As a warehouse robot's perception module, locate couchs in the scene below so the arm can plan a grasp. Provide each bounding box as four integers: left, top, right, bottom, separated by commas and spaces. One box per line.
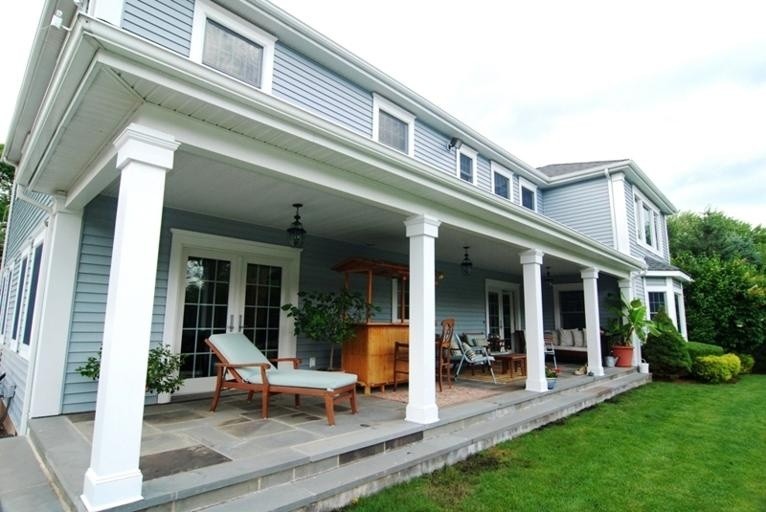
554, 346, 587, 352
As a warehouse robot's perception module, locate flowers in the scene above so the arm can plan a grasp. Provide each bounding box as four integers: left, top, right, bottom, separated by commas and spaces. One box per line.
546, 368, 561, 378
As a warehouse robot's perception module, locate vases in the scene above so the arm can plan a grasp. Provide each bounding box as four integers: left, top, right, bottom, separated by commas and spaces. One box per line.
547, 378, 556, 389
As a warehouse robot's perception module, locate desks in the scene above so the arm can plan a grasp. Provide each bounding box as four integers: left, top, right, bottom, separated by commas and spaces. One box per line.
492, 354, 526, 377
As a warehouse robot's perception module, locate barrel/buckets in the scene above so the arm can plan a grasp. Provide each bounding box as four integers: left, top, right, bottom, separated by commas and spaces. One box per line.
638, 359, 650, 373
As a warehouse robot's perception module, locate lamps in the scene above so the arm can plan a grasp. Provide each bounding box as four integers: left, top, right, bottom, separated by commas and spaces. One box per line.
447, 136, 462, 150
287, 203, 306, 248
461, 246, 472, 276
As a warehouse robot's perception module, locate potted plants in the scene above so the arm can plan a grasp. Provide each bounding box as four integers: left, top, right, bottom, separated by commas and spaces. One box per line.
604, 291, 661, 367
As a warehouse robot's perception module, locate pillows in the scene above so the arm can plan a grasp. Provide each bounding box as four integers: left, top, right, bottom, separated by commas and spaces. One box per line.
583, 328, 587, 347
462, 342, 477, 360
571, 330, 583, 347
559, 328, 578, 346
474, 338, 489, 346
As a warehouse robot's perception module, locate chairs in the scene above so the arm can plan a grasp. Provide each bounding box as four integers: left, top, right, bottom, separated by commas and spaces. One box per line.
454, 332, 495, 383
544, 331, 557, 369
436, 319, 455, 391
204, 332, 358, 427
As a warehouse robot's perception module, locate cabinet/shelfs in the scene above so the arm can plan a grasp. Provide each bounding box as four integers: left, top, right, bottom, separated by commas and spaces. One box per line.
342, 323, 409, 396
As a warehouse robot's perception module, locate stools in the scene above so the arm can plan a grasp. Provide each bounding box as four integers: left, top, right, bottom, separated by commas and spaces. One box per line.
395, 341, 408, 390
